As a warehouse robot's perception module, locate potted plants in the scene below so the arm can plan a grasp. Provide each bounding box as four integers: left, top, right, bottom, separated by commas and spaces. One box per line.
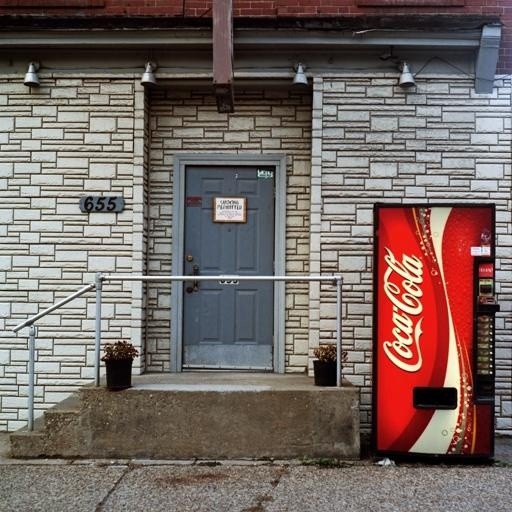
101, 340, 139, 389
312, 343, 349, 386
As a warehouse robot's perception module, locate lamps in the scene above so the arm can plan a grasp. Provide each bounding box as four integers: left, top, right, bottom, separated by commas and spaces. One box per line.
291, 59, 415, 89
23, 56, 160, 89
